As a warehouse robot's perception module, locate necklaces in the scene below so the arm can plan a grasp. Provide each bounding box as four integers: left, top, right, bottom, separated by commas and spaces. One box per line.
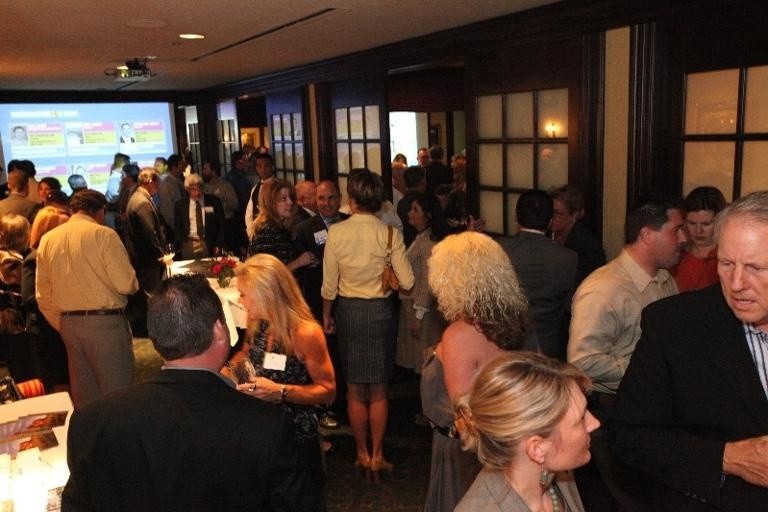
548, 483, 559, 512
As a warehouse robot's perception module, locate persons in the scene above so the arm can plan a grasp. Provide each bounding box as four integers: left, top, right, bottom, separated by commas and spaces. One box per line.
21, 206, 72, 391
419, 231, 542, 512
221, 253, 338, 511
104, 153, 130, 204
225, 152, 252, 255
391, 145, 466, 215
67, 175, 88, 199
107, 165, 140, 269
244, 154, 275, 244
339, 199, 403, 234
320, 168, 415, 472
1, 212, 33, 383
0, 160, 19, 200
31, 178, 72, 252
159, 154, 186, 234
394, 192, 451, 428
174, 174, 224, 261
547, 185, 604, 289
245, 178, 336, 455
16, 160, 40, 202
490, 190, 579, 361
0, 170, 43, 223
669, 186, 727, 295
600, 191, 768, 512
126, 168, 175, 336
447, 352, 599, 512
201, 160, 240, 256
61, 275, 326, 511
152, 158, 168, 206
293, 180, 351, 429
566, 197, 687, 396
35, 188, 140, 410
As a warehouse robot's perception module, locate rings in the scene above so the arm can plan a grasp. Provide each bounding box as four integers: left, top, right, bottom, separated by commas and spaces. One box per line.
253, 384, 256, 390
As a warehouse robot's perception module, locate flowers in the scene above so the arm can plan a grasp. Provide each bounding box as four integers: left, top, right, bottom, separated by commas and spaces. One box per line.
208, 248, 238, 289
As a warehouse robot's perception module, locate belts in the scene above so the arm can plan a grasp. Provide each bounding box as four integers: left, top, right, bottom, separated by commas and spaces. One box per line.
56, 309, 124, 318
430, 423, 461, 440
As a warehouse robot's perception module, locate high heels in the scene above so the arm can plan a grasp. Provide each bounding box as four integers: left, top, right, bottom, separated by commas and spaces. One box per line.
369, 461, 396, 486
354, 461, 369, 487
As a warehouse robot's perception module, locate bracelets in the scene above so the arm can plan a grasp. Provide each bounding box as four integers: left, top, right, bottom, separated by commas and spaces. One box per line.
281, 383, 288, 405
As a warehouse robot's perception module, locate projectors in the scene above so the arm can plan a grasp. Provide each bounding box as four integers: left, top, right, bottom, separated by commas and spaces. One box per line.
114, 65, 151, 81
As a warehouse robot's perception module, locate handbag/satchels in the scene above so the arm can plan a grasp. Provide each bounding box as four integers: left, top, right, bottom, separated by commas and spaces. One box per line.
380, 224, 400, 291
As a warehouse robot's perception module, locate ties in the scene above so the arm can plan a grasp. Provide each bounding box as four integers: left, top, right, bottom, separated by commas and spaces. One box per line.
195, 201, 203, 238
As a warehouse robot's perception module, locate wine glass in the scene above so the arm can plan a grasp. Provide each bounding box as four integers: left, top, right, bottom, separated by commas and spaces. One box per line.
423, 346, 436, 363
165, 244, 175, 265
230, 358, 256, 393
213, 247, 223, 262
